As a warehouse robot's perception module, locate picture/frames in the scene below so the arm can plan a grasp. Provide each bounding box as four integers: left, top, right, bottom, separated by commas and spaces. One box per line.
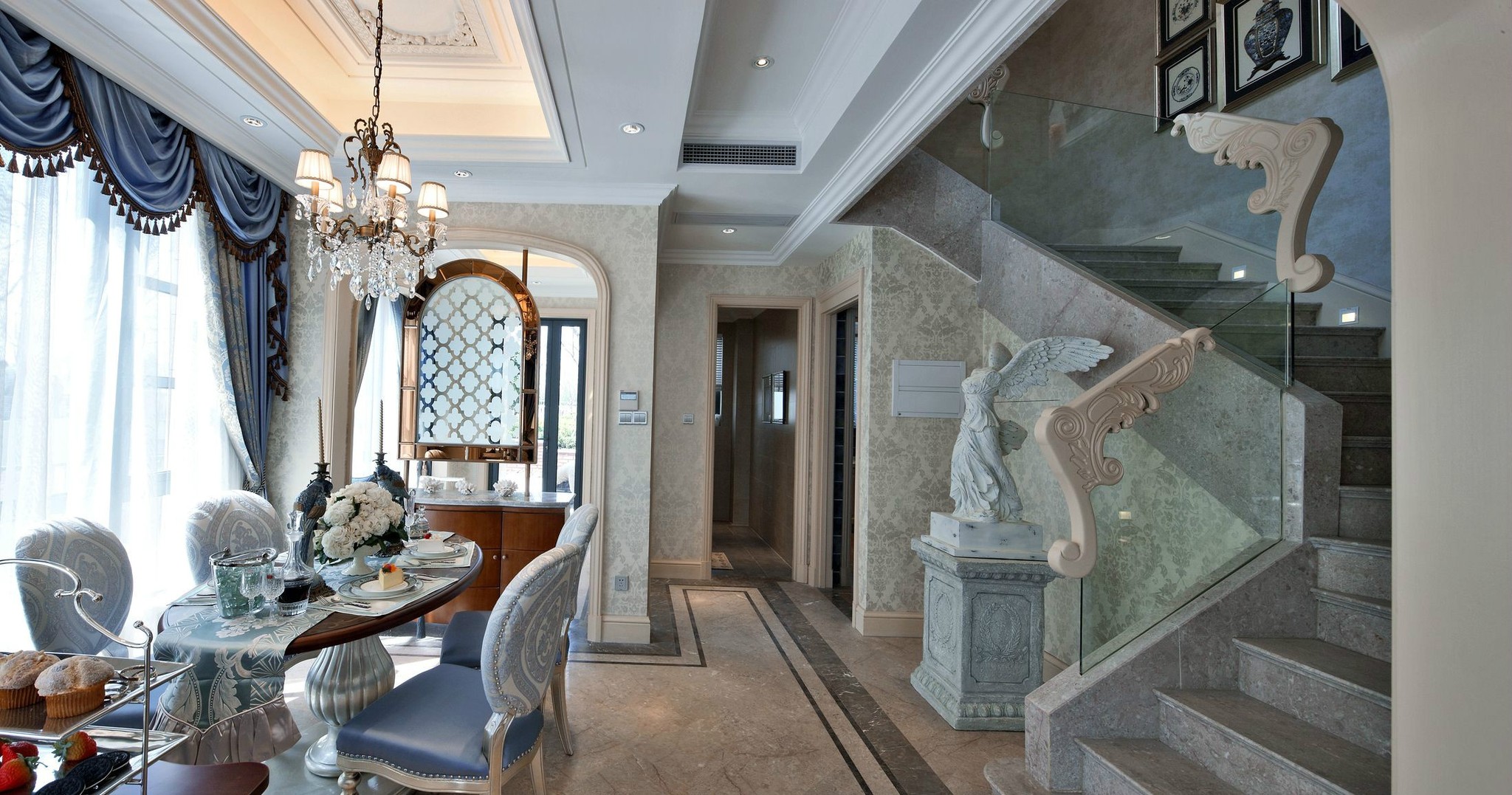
1153, 28, 1217, 133
1329, 0, 1377, 83
1154, 0, 1216, 58
1215, 0, 1327, 113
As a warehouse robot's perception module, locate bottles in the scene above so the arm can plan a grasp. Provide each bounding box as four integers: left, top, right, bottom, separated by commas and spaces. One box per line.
414, 504, 430, 539
274, 511, 314, 615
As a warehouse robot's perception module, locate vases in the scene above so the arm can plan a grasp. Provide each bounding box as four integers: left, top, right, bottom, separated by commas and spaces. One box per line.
340, 545, 381, 576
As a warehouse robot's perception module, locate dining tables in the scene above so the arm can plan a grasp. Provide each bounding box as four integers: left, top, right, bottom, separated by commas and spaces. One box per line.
149, 530, 482, 778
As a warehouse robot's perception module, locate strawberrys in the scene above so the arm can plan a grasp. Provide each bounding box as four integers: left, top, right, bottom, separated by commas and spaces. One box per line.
0, 737, 39, 793
53, 732, 97, 763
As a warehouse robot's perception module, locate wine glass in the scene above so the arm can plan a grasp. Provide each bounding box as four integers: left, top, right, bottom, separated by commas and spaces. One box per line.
238, 569, 262, 625
261, 566, 286, 627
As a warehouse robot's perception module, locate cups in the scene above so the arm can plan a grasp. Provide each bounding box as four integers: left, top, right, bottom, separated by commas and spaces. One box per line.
404, 495, 416, 544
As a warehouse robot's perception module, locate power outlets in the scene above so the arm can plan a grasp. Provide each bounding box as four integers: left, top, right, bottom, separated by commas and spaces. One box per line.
615, 575, 629, 590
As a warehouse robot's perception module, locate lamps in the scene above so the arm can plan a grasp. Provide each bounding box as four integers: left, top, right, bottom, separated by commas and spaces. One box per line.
292, 0, 450, 313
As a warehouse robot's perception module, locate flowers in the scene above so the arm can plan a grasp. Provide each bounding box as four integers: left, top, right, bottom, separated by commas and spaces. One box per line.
313, 481, 411, 564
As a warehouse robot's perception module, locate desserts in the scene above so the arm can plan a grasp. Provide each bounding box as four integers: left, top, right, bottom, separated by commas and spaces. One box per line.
379, 563, 403, 590
417, 533, 445, 552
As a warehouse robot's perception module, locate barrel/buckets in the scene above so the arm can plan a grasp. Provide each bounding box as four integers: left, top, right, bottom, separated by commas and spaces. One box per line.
208, 548, 278, 619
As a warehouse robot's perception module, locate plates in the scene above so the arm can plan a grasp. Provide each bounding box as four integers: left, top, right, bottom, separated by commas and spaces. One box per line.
338, 574, 424, 600
400, 542, 467, 559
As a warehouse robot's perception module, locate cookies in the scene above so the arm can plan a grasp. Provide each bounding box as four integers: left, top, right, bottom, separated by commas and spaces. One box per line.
36, 750, 130, 795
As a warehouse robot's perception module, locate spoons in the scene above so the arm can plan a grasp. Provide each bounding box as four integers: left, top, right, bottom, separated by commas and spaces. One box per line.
418, 559, 456, 564
331, 597, 370, 605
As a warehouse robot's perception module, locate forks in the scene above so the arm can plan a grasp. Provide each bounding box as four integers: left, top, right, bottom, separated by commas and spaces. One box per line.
404, 560, 455, 568
316, 595, 372, 609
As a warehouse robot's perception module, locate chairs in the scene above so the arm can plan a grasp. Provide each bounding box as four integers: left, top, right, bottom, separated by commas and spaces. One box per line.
183, 489, 287, 586
14, 517, 171, 730
337, 544, 577, 795
439, 503, 600, 755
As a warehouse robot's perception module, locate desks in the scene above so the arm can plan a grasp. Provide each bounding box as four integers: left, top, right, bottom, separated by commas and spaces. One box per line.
102, 760, 271, 795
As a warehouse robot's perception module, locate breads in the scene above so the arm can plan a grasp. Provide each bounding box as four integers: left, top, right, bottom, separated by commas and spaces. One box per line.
1, 650, 114, 717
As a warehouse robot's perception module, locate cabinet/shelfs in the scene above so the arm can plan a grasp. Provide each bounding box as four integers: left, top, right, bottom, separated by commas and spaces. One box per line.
406, 488, 577, 625
0, 558, 196, 795
